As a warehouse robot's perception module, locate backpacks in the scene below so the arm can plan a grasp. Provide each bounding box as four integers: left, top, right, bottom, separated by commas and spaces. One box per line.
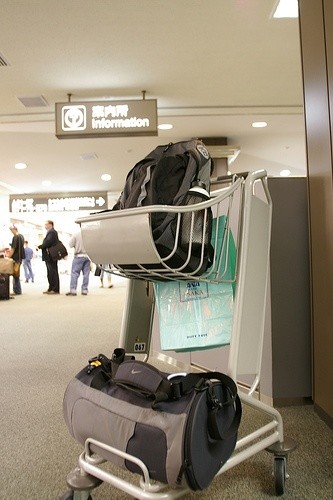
90, 138, 215, 282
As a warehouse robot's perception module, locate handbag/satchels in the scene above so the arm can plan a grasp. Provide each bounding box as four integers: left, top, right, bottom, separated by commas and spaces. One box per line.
61, 349, 242, 493
12, 261, 21, 278
47, 241, 68, 261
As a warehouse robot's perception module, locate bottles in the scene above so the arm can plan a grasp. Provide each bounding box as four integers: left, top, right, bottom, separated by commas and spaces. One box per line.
181, 181, 210, 247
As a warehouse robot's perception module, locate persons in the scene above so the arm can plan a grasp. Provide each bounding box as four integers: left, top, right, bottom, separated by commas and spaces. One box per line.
100, 264, 113, 288
23, 241, 34, 283
66, 223, 90, 295
9, 226, 25, 294
36, 221, 59, 294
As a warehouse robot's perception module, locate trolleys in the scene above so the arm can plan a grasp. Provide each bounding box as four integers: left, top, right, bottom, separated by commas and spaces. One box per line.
65, 169, 298, 500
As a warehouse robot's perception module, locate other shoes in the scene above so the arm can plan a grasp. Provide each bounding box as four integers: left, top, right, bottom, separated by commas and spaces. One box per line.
43, 289, 50, 294
65, 291, 77, 296
48, 290, 60, 294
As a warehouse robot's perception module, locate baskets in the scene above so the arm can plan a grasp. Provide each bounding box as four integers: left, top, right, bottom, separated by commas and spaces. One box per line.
76, 176, 243, 283
